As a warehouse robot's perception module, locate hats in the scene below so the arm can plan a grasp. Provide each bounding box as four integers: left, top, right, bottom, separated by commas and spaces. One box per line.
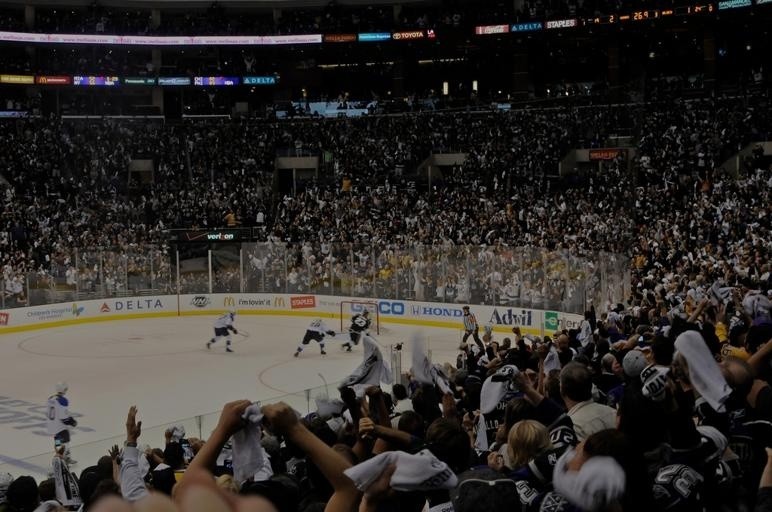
622, 350, 648, 377
363, 309, 369, 314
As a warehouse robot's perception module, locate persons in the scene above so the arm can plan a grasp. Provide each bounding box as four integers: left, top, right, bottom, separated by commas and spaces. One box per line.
0, 2, 772, 511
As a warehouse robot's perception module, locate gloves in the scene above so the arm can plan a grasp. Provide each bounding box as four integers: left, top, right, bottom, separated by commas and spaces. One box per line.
326, 330, 336, 337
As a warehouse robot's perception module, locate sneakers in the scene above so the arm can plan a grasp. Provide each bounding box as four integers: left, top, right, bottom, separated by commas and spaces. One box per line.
206, 342, 211, 350
320, 351, 327, 354
294, 352, 300, 357
342, 343, 350, 348
65, 457, 78, 465
346, 346, 353, 352
225, 348, 233, 352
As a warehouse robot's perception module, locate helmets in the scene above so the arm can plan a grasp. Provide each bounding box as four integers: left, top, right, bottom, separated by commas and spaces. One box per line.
230, 310, 236, 316
55, 380, 69, 396
462, 306, 470, 310
